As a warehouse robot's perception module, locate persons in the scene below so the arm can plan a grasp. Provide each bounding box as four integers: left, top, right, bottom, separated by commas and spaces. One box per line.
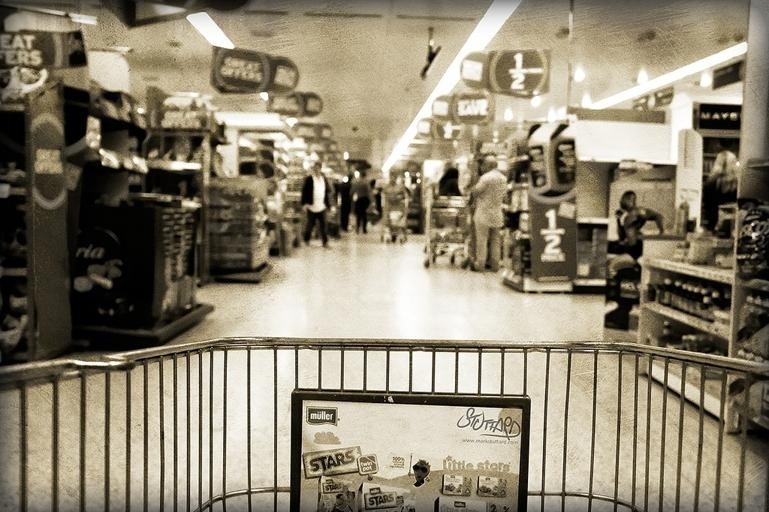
301, 161, 332, 250
327, 150, 568, 273
606, 190, 663, 301
702, 149, 741, 237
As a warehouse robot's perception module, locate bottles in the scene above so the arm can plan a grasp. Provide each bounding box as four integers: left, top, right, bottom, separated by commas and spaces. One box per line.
660, 276, 762, 331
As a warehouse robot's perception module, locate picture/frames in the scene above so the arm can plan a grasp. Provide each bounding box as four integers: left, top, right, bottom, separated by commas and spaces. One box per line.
289, 387, 532, 512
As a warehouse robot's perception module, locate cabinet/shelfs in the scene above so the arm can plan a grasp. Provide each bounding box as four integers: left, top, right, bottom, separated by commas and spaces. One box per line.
1, 81, 274, 362
634, 160, 769, 435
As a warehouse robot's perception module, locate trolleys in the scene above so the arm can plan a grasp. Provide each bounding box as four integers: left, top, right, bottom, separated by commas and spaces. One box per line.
381, 206, 408, 244
